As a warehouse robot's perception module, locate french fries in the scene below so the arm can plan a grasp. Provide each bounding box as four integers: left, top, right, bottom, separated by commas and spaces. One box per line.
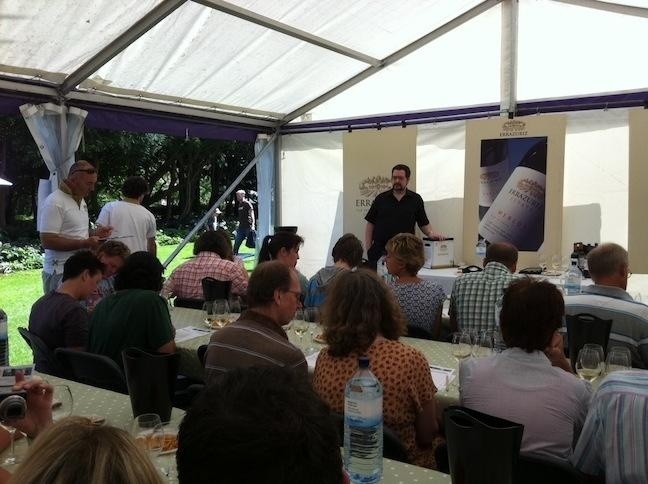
159, 438, 176, 451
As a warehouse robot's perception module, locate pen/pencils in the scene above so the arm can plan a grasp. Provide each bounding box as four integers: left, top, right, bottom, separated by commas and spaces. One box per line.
97, 222, 119, 233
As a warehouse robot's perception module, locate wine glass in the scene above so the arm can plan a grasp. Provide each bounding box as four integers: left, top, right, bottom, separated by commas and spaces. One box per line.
2, 384, 74, 464
294, 303, 326, 354
538, 251, 570, 278
202, 295, 241, 339
130, 411, 181, 482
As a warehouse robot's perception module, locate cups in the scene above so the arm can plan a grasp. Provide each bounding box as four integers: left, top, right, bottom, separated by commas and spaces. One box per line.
576, 342, 631, 382
451, 328, 505, 365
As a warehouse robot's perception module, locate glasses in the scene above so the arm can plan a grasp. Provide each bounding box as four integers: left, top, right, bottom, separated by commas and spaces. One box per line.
72, 168, 98, 175
625, 261, 632, 279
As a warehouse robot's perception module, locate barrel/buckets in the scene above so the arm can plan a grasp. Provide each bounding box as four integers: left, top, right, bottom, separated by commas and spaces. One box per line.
200, 275, 233, 316
120, 341, 184, 425
564, 309, 612, 373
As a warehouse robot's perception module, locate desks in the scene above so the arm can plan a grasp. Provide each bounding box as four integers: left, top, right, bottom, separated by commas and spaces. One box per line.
167, 261, 647, 451
1, 372, 452, 484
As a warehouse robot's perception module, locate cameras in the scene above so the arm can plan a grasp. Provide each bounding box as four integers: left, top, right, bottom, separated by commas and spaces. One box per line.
0, 389, 28, 420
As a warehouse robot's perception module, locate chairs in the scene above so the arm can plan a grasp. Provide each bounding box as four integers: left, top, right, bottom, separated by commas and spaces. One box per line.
16, 327, 54, 375
53, 348, 197, 409
434, 443, 590, 484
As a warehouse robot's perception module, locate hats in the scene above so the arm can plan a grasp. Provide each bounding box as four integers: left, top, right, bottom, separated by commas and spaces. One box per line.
236, 190, 246, 194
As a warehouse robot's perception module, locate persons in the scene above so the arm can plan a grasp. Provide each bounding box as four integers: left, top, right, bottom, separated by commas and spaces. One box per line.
232, 189, 257, 256
364, 164, 445, 272
93, 175, 157, 257
39, 160, 114, 296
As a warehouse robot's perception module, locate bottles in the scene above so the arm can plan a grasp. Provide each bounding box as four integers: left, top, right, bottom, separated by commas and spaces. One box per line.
0, 307, 10, 367
343, 353, 383, 480
474, 237, 487, 269
479, 138, 544, 250
564, 260, 582, 295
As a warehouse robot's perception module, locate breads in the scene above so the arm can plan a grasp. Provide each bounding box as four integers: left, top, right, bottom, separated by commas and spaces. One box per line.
136, 435, 160, 449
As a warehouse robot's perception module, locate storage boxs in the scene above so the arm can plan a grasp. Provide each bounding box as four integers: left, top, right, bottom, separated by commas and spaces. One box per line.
422, 233, 457, 269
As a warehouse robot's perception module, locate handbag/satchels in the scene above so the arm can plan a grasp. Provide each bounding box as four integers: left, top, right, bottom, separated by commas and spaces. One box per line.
246, 230, 256, 248
